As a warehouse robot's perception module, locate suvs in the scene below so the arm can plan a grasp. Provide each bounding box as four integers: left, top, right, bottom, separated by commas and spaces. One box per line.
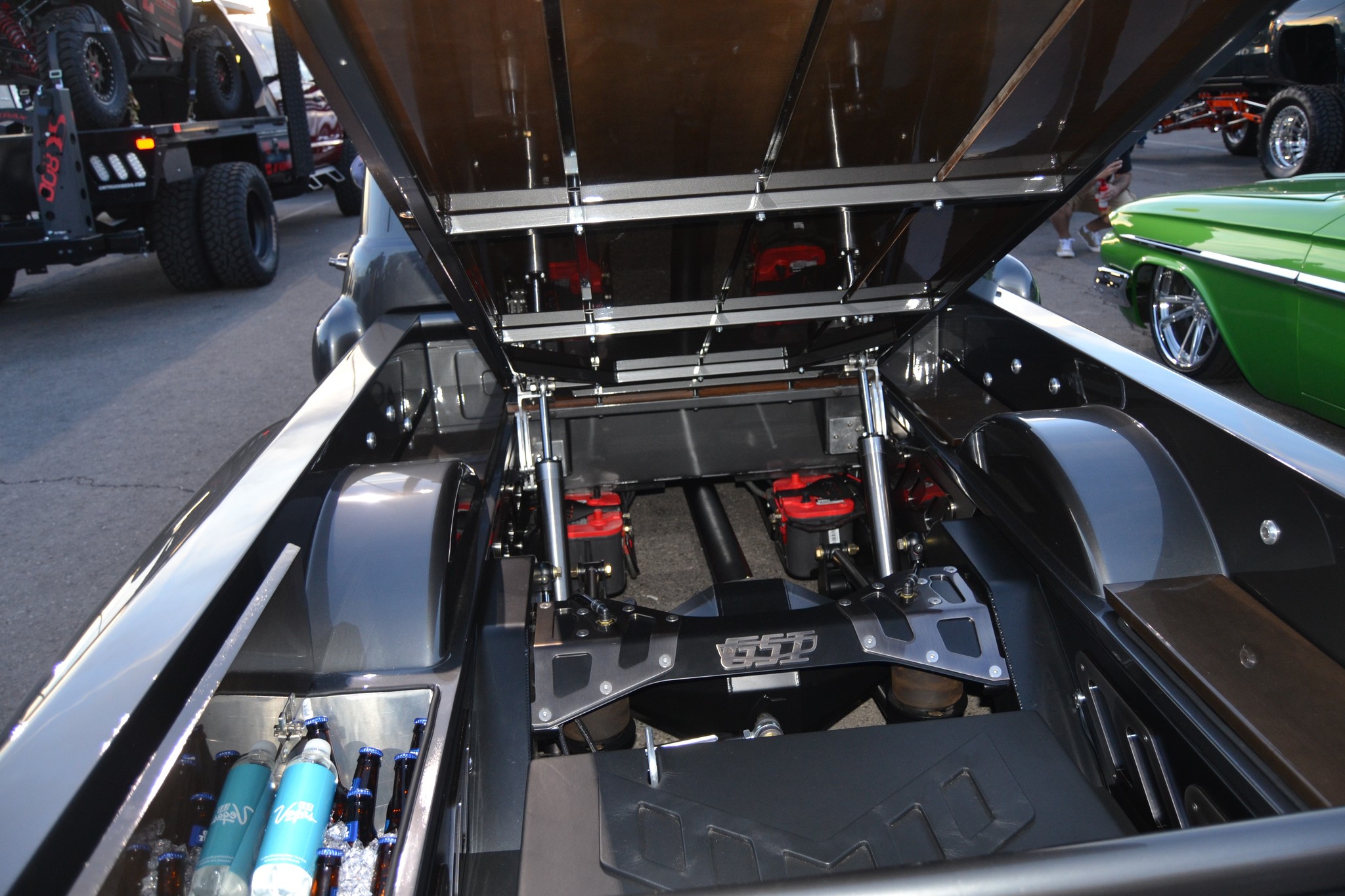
1152, 16, 1345, 180
0, 0, 366, 300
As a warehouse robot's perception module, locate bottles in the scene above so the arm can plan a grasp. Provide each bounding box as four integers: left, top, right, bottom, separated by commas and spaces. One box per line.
252, 712, 429, 896
118, 722, 278, 895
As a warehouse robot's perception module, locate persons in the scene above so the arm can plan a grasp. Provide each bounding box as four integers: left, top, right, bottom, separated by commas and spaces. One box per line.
1136, 133, 1147, 148
1045, 142, 1140, 258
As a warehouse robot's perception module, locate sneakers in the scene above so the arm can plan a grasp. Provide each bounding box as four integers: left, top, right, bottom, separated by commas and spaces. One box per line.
1078, 225, 1102, 252
1057, 237, 1075, 256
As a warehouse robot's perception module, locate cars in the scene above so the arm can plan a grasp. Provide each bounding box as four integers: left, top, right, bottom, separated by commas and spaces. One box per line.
1094, 172, 1344, 430
